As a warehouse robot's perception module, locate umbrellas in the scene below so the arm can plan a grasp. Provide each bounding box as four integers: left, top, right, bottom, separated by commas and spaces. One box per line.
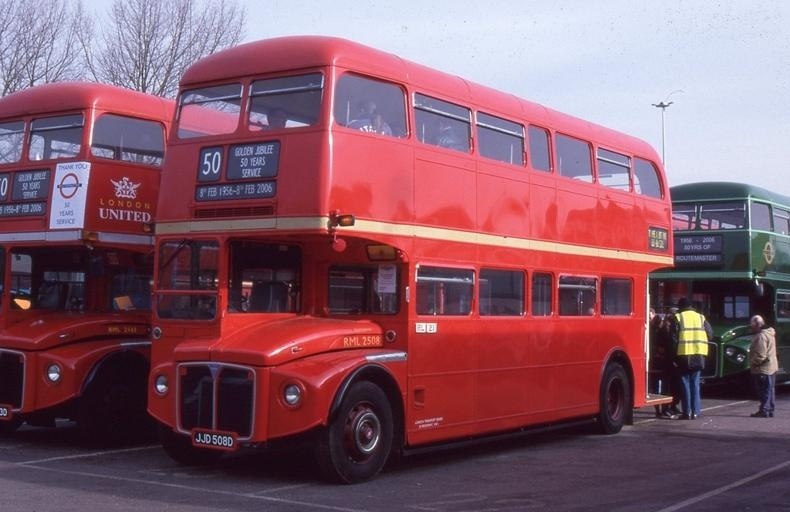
678, 411, 699, 420
750, 409, 774, 417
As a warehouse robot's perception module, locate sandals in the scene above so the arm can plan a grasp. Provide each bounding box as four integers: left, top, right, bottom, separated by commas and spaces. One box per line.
646, 180, 789, 396
142, 32, 675, 486
1, 79, 267, 445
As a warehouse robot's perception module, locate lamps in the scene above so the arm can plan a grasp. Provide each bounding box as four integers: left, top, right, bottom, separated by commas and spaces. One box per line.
248, 279, 288, 313
34, 281, 72, 311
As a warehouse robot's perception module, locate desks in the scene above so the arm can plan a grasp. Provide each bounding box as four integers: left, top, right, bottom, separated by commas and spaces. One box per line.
651, 101, 674, 169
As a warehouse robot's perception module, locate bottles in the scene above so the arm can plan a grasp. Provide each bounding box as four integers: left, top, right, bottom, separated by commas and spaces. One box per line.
676, 297, 693, 308
750, 315, 765, 328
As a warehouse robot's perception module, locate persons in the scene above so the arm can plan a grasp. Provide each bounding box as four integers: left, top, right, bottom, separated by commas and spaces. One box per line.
261, 108, 288, 131
433, 118, 466, 152
348, 98, 393, 137
749, 314, 779, 418
649, 297, 714, 420
